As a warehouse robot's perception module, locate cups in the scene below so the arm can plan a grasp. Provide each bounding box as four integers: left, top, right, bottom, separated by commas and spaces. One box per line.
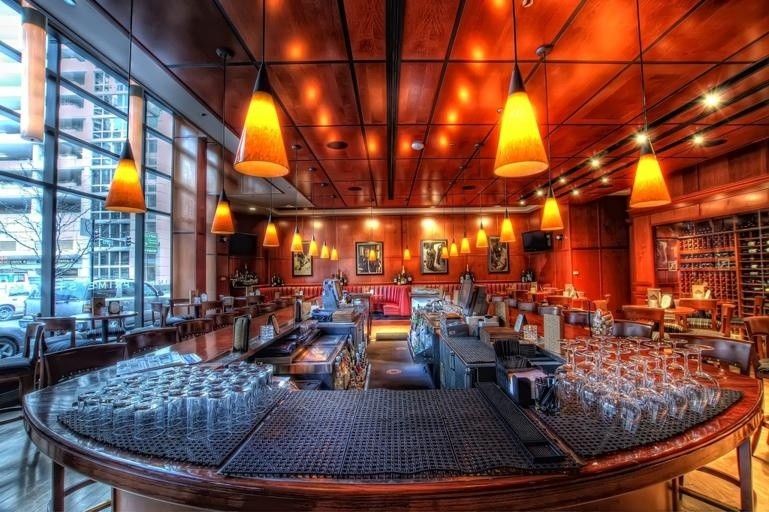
75, 361, 276, 443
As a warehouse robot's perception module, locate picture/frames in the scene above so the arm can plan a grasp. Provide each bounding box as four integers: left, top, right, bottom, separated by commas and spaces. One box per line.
487, 235, 510, 274
355, 241, 384, 276
420, 238, 449, 275
291, 240, 314, 277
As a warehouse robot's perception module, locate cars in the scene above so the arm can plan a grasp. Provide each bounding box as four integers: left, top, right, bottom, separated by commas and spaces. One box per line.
0, 266, 170, 359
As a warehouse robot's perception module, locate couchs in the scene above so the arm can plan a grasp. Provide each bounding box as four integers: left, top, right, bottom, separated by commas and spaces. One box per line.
322, 286, 410, 317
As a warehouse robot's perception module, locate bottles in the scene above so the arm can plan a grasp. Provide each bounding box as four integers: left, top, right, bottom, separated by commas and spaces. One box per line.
393, 267, 413, 285
737, 214, 769, 294
458, 264, 475, 285
271, 272, 285, 287
330, 268, 347, 285
233, 263, 259, 286
293, 296, 302, 322
521, 266, 535, 284
311, 299, 320, 317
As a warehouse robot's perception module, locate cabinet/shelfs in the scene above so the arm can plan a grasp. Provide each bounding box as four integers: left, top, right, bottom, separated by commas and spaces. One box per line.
675, 208, 769, 316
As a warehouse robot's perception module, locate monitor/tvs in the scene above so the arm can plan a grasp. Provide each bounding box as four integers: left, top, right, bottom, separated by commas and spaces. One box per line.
522, 230, 552, 252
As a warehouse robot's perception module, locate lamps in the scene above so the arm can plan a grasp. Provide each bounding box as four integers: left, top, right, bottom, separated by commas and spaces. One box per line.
232, 1, 291, 178
437, 142, 489, 260
105, 1, 150, 215
127, 84, 145, 182
499, 176, 517, 243
19, 0, 48, 145
403, 198, 412, 260
210, 47, 236, 236
492, 0, 550, 178
627, 0, 672, 209
367, 198, 377, 263
288, 143, 339, 260
262, 176, 281, 248
534, 43, 565, 231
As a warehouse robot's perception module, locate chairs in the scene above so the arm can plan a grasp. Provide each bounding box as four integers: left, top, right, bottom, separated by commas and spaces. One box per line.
0, 286, 322, 511
485, 285, 769, 511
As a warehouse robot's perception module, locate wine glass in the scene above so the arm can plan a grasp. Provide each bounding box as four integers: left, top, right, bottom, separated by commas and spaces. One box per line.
535, 334, 723, 436
